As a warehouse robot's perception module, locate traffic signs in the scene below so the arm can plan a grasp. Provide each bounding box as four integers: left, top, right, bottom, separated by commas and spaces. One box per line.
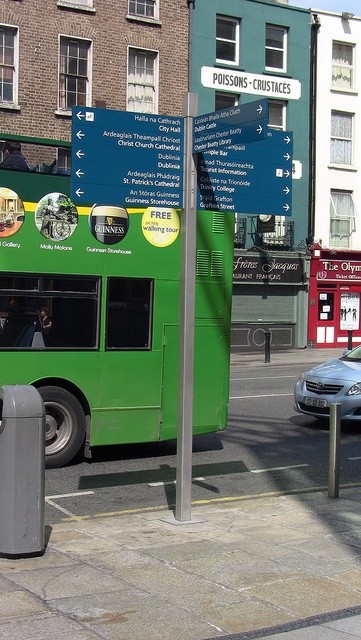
72, 106, 184, 208
196, 129, 293, 215
192, 98, 269, 153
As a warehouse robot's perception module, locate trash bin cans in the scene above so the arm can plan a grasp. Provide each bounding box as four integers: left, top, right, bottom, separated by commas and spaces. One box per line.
0, 384, 45, 556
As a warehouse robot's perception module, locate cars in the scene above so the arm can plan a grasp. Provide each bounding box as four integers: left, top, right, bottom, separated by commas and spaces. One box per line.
293, 343, 361, 424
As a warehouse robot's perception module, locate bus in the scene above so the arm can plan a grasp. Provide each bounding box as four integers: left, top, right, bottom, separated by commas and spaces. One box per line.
0, 131, 235, 467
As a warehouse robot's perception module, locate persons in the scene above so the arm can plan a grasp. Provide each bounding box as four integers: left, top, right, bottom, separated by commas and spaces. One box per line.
38, 198, 57, 236
0, 138, 31, 174
39, 305, 52, 344
340, 304, 359, 324
